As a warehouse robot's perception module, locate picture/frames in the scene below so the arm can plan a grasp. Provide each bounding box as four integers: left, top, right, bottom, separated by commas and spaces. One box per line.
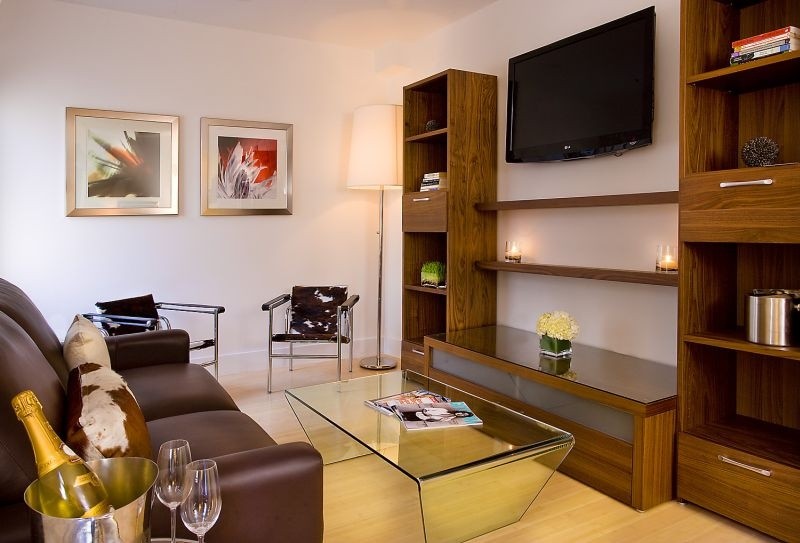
65, 107, 179, 215
201, 117, 293, 216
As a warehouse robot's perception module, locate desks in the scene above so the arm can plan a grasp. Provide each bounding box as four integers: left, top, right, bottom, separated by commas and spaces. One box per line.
424, 325, 678, 513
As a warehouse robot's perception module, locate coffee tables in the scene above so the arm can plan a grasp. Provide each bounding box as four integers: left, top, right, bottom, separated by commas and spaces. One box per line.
284, 369, 575, 543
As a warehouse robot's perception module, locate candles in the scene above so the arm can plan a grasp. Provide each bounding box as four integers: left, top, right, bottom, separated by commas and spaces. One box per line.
657, 256, 678, 269
505, 246, 521, 260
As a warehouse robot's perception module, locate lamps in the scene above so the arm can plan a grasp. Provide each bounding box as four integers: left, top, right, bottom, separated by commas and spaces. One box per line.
346, 103, 403, 370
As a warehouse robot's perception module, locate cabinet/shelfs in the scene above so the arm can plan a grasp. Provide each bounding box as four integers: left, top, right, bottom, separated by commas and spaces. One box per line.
474, 189, 679, 286
676, 0, 799, 543
401, 69, 498, 383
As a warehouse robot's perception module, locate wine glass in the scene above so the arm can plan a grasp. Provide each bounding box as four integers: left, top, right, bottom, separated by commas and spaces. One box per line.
178, 459, 222, 543
154, 439, 194, 543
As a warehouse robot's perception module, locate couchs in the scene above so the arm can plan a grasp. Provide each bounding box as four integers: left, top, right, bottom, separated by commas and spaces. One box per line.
0, 277, 323, 543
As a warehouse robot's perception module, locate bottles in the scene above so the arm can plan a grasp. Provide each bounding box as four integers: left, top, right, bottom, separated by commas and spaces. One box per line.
11, 390, 111, 519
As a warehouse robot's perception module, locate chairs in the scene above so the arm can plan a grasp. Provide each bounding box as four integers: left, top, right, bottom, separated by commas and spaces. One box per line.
262, 284, 360, 394
83, 294, 225, 374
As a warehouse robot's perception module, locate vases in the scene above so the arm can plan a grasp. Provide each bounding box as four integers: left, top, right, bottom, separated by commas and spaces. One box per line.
540, 334, 572, 357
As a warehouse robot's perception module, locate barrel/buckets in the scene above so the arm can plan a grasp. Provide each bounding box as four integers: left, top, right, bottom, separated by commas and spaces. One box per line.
23, 456, 160, 543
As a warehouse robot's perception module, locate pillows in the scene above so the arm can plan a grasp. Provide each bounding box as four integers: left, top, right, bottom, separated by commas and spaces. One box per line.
66, 364, 154, 460
63, 314, 111, 370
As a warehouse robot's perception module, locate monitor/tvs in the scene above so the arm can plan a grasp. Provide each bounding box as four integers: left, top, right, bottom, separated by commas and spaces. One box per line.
506, 6, 654, 163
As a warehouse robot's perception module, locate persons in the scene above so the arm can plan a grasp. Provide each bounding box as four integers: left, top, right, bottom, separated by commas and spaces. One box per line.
401, 407, 474, 422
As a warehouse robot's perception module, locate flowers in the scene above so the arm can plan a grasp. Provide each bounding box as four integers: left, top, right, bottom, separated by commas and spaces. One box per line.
537, 311, 580, 342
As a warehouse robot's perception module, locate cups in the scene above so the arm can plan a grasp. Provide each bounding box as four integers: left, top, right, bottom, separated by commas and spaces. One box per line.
656, 243, 679, 274
504, 240, 522, 264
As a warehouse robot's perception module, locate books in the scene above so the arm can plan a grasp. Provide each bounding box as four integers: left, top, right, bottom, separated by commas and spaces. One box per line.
390, 401, 483, 432
364, 387, 451, 417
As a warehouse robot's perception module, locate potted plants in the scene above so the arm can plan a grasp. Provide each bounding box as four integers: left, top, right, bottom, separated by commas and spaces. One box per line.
421, 261, 446, 289
